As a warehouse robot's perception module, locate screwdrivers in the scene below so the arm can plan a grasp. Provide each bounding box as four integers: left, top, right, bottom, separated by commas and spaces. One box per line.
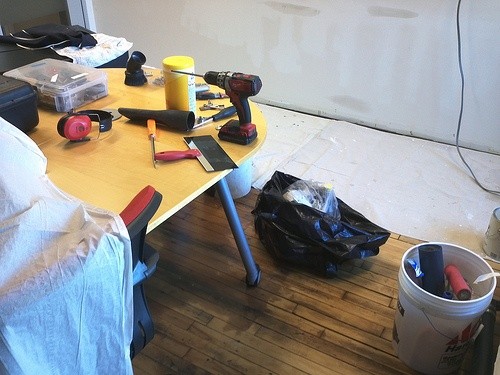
147, 119, 158, 169
193, 106, 237, 130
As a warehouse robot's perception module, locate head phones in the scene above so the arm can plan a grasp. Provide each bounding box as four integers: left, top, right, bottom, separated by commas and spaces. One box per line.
57, 109, 113, 142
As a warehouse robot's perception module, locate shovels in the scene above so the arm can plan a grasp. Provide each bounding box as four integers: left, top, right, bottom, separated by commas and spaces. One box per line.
154, 135, 239, 172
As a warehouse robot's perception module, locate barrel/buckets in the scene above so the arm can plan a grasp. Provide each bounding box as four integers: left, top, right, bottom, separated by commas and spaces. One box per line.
225, 160, 252, 199
483, 208, 500, 261
392, 242, 497, 375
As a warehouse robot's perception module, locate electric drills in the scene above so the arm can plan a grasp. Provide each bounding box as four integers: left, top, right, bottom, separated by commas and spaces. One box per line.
171, 68, 262, 146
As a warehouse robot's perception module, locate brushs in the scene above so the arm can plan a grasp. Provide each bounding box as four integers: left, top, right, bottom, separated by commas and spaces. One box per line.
444, 265, 473, 301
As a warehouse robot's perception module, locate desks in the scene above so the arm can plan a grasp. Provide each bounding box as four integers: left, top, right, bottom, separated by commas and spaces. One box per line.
27, 67, 269, 289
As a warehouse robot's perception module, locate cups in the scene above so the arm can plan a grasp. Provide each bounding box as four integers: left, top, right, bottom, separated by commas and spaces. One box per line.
162, 56, 195, 114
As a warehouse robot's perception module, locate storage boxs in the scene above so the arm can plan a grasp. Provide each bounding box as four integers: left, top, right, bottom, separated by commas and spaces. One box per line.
0, 74, 40, 134
3, 57, 108, 113
